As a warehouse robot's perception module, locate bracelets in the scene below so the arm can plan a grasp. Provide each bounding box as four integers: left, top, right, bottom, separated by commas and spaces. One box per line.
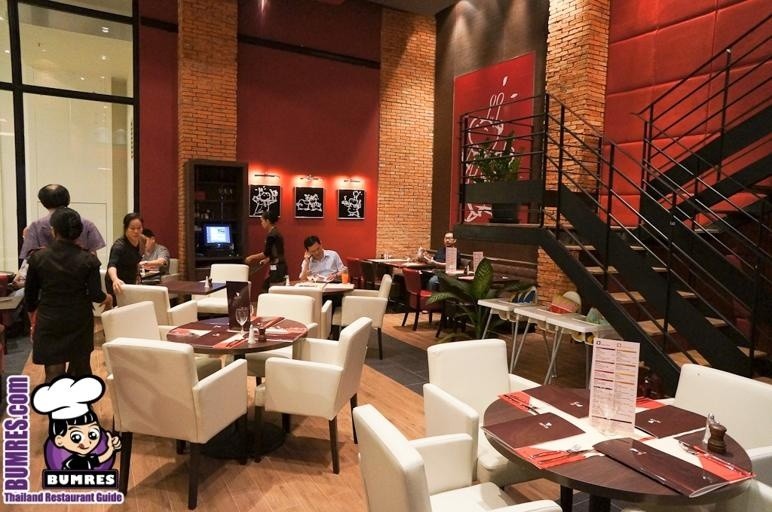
29, 325, 34, 329
333, 273, 338, 279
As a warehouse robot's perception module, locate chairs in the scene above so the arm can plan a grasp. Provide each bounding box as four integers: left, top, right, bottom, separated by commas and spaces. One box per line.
100, 258, 772, 512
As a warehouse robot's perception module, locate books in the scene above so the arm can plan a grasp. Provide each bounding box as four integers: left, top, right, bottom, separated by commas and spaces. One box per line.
190, 329, 237, 348
522, 383, 589, 418
634, 404, 708, 438
250, 316, 282, 329
480, 412, 585, 450
178, 321, 229, 330
592, 437, 729, 499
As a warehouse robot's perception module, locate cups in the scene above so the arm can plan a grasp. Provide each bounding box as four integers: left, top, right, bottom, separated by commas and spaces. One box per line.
341, 266, 349, 284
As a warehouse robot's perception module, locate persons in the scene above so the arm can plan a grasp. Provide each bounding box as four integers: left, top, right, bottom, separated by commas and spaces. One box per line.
421, 231, 460, 314
138, 229, 170, 274
245, 211, 288, 293
20, 184, 106, 300
298, 235, 348, 339
22, 208, 114, 385
105, 213, 143, 307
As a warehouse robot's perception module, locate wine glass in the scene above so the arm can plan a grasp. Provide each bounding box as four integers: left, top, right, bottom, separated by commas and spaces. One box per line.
305, 270, 320, 288
234, 307, 248, 336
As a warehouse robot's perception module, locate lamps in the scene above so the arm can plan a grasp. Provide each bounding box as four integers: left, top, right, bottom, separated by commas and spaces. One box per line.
342, 175, 362, 184
253, 167, 281, 179
298, 170, 323, 181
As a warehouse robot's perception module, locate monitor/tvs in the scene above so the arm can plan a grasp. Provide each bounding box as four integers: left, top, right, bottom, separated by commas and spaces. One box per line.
203, 223, 233, 247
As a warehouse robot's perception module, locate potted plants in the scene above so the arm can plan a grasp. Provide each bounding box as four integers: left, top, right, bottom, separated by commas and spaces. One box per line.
466, 129, 525, 225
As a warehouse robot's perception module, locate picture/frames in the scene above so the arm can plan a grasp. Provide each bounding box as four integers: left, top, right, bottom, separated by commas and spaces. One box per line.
248, 183, 283, 219
336, 188, 365, 221
293, 186, 325, 219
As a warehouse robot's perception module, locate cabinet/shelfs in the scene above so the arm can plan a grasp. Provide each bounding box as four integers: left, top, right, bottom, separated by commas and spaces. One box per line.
183, 156, 250, 282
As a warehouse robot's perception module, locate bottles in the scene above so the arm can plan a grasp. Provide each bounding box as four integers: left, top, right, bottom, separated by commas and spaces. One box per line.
464, 264, 470, 276
247, 323, 267, 344
701, 412, 726, 452
204, 275, 212, 288
284, 275, 291, 285
